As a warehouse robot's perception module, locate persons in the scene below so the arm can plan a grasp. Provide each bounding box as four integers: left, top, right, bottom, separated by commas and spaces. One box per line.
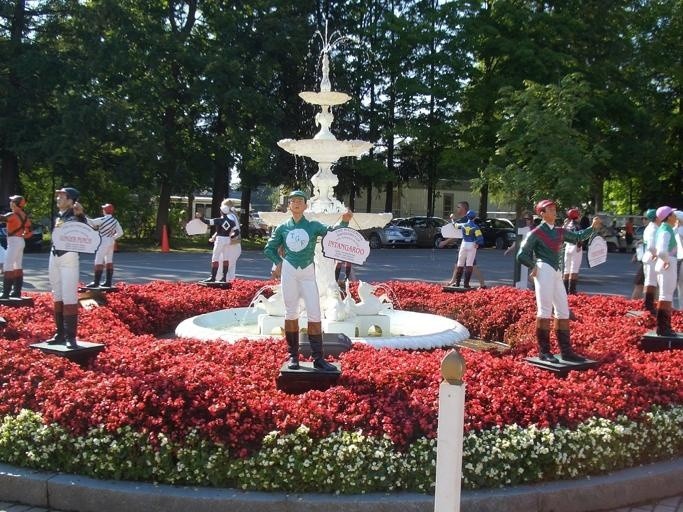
439, 201, 487, 289
0, 220, 12, 280
448, 209, 484, 288
208, 200, 241, 283
46, 187, 85, 349
504, 207, 682, 310
194, 206, 240, 283
652, 206, 677, 336
0, 192, 34, 298
82, 201, 125, 288
263, 191, 354, 372
517, 198, 602, 364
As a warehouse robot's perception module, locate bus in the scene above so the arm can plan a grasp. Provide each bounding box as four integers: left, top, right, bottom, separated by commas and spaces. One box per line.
153, 195, 268, 239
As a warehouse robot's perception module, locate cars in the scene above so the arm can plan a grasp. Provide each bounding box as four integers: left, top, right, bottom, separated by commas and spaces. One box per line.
533, 218, 581, 232
357, 218, 418, 250
604, 225, 645, 252
432, 217, 516, 248
0, 221, 42, 250
396, 216, 457, 249
512, 216, 533, 228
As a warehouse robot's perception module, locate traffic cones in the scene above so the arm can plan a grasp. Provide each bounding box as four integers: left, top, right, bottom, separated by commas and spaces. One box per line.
160, 224, 170, 253
